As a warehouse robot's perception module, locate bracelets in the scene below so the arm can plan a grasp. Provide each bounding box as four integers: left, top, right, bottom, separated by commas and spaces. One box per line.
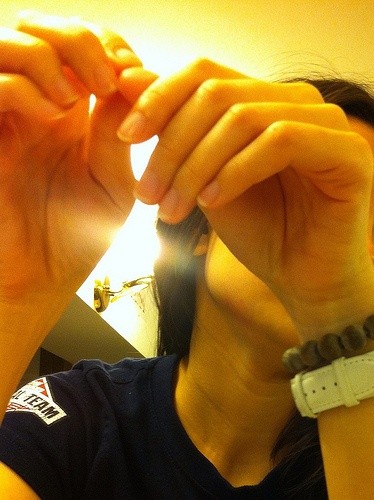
290, 352, 374, 419
282, 313, 374, 373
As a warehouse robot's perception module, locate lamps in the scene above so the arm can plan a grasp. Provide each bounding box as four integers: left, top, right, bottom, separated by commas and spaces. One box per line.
93, 258, 155, 313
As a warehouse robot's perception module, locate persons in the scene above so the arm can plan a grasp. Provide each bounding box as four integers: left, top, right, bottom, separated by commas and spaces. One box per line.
0, 15, 374, 499
